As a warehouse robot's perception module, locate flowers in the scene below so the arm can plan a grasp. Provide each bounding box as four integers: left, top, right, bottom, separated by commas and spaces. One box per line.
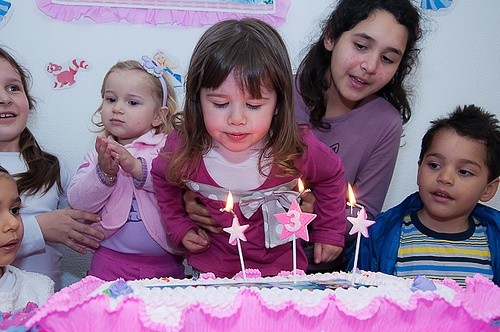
102, 279, 132, 300
409, 274, 437, 292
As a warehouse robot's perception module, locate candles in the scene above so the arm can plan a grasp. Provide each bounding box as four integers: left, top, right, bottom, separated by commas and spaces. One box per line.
347, 181, 376, 285
219, 190, 247, 280
274, 177, 317, 283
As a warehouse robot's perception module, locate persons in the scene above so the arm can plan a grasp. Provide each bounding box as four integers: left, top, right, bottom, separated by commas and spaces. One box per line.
68, 55, 186, 279
182, 0, 423, 276
344, 104, 500, 287
0, 165, 54, 313
150, 19, 347, 279
0, 47, 104, 291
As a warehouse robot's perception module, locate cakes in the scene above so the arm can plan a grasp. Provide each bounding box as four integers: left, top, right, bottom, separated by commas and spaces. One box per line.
84, 268, 456, 326
